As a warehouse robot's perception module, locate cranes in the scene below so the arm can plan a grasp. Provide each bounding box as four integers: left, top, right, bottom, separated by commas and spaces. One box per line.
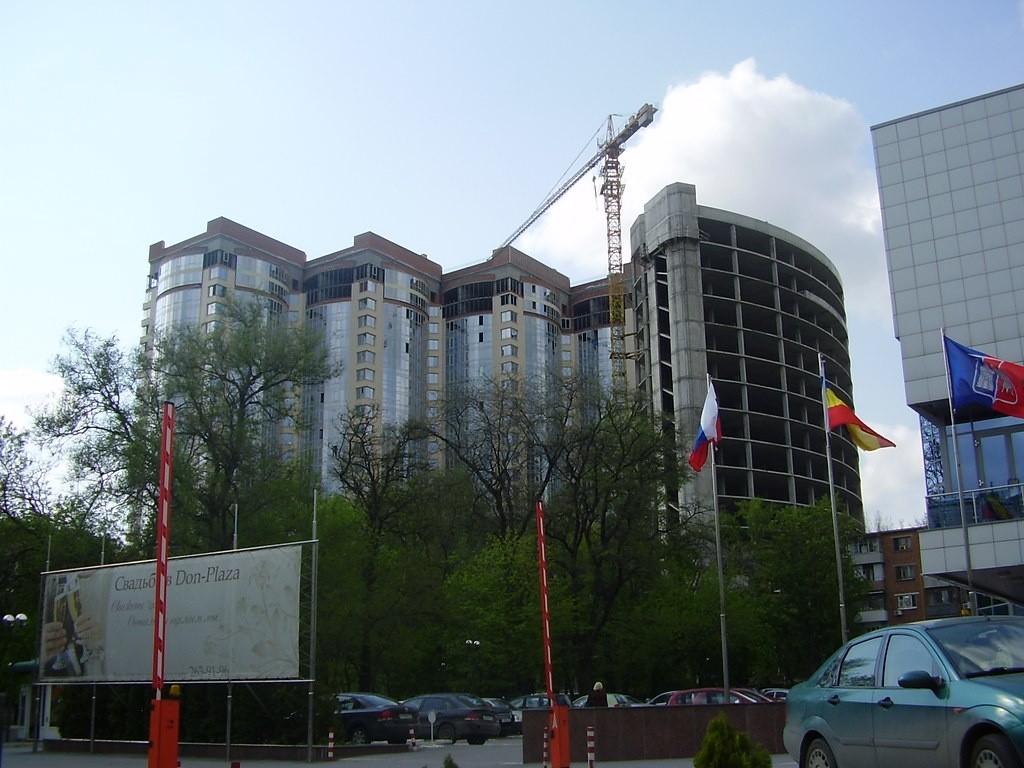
478, 103, 661, 436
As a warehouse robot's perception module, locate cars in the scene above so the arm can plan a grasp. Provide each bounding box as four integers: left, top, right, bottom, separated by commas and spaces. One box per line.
467, 687, 786, 738
398, 693, 501, 746
284, 691, 420, 745
782, 613, 1024, 768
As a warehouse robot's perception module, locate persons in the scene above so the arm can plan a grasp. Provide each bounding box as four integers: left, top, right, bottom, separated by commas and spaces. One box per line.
41, 605, 103, 677
587, 682, 607, 707
557, 689, 572, 708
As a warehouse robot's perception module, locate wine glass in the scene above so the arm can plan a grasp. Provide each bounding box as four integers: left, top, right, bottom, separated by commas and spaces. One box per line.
67, 583, 88, 664
52, 584, 67, 671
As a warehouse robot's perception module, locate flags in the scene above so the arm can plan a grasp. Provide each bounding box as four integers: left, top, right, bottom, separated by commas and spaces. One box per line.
688, 377, 722, 472
944, 336, 1024, 419
819, 361, 896, 451
979, 484, 1010, 522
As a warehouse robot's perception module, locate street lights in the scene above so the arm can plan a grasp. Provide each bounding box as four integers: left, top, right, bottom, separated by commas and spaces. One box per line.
3, 612, 27, 743
223, 503, 240, 760
